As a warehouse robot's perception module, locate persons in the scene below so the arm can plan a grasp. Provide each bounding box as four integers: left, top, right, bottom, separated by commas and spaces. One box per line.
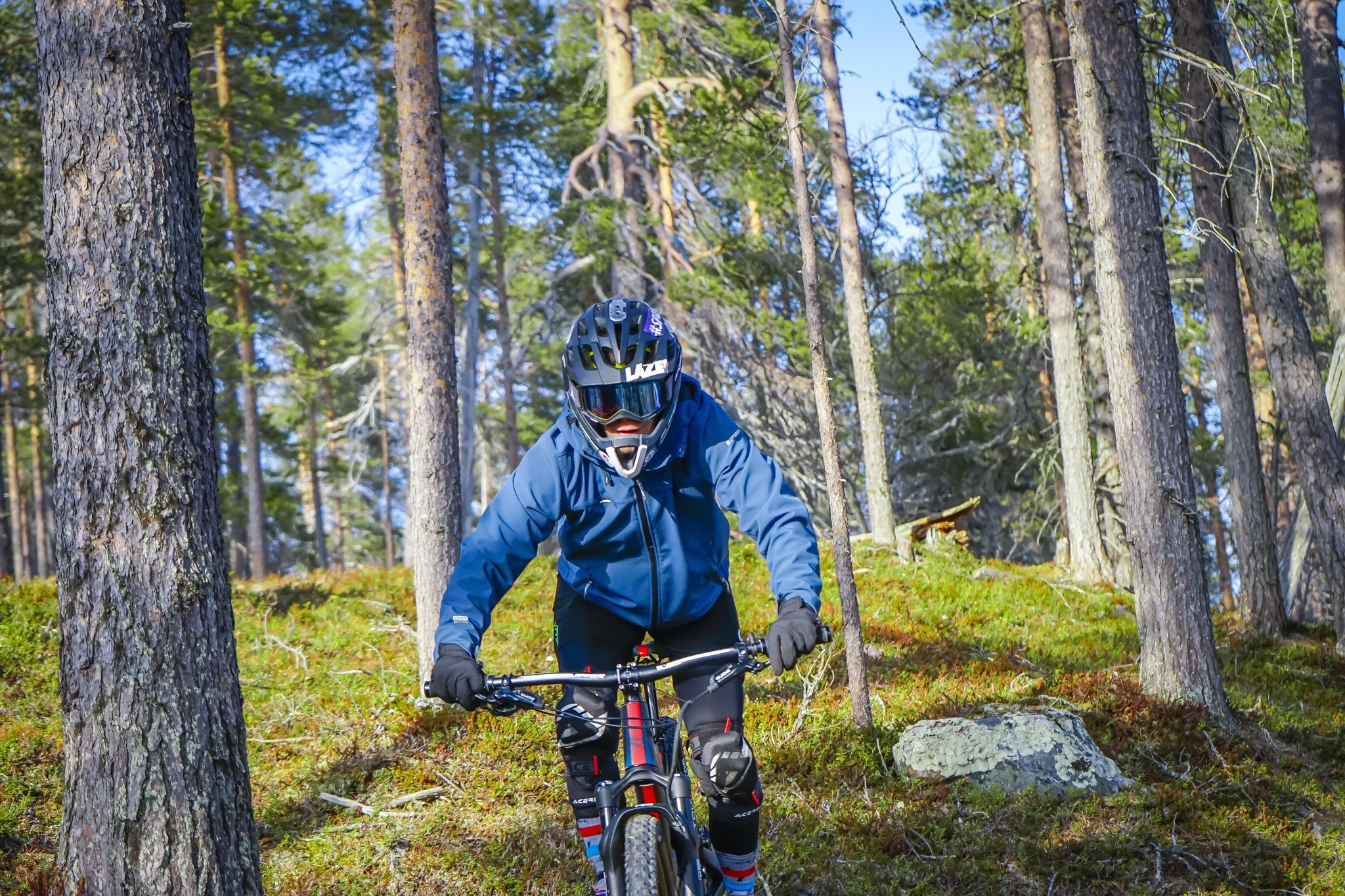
430, 300, 822, 896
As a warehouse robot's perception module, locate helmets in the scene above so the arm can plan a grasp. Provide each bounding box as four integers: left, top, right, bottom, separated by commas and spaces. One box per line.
561, 298, 683, 479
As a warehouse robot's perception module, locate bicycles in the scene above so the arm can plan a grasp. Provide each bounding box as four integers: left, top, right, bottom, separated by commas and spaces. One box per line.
424, 625, 835, 896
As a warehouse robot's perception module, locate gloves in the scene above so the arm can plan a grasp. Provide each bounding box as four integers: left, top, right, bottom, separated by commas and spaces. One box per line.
767, 595, 818, 676
430, 642, 483, 712
